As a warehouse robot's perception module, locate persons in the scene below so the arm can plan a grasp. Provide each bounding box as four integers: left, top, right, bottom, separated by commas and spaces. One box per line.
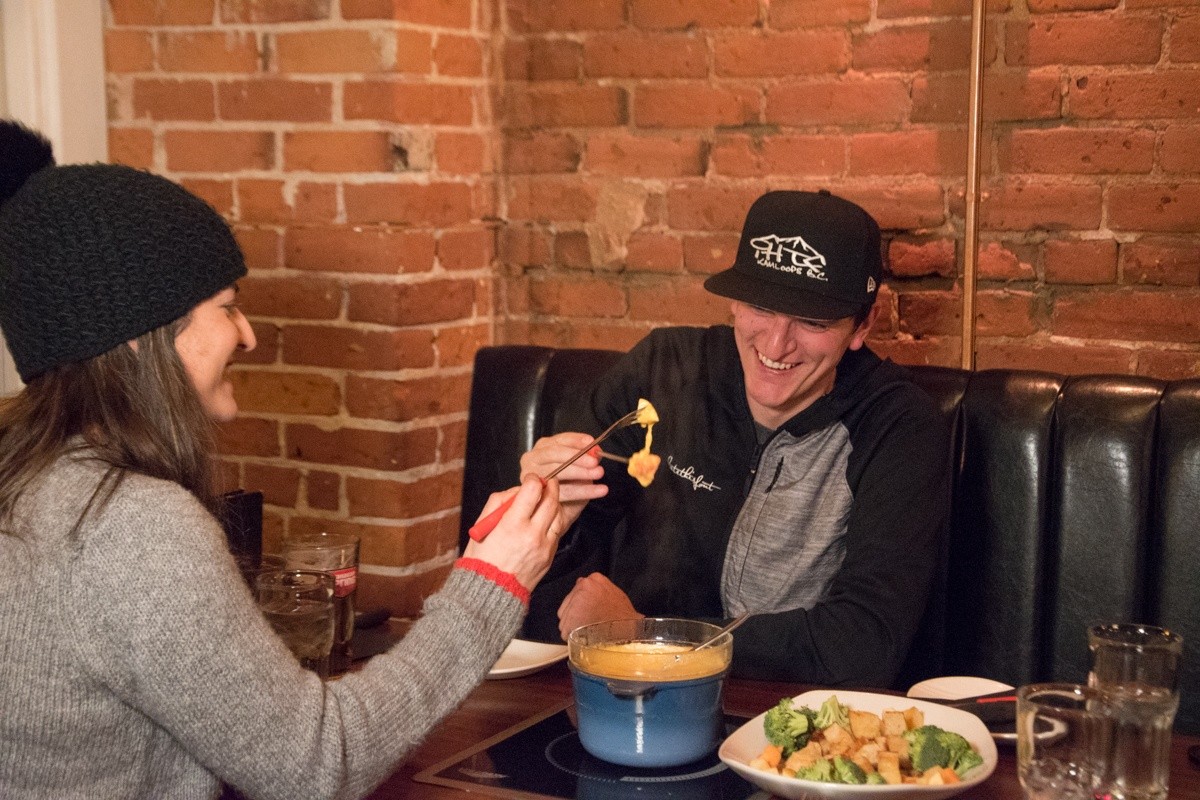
519, 188, 950, 690
0, 118, 566, 800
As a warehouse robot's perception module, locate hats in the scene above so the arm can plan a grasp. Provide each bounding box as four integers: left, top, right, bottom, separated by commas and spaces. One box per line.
704, 189, 883, 320
0, 119, 248, 384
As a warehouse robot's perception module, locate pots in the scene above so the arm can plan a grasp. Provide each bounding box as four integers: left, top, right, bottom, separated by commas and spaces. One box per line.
566, 617, 732, 768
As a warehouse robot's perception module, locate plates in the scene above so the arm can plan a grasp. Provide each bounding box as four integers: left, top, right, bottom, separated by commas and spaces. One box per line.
905, 677, 1017, 700
718, 689, 998, 799
486, 637, 569, 681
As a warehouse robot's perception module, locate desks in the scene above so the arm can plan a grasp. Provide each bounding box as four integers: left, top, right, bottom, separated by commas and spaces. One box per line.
328, 617, 1200, 800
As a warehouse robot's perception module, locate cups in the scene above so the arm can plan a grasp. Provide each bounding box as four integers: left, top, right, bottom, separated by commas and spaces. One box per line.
252, 571, 337, 682
1087, 625, 1184, 800
281, 533, 362, 655
1016, 685, 1124, 799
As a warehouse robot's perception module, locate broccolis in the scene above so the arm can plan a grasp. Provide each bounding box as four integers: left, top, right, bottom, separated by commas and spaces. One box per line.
763, 694, 983, 784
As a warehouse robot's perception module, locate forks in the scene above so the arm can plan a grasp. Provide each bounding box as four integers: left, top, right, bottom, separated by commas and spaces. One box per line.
468, 407, 642, 543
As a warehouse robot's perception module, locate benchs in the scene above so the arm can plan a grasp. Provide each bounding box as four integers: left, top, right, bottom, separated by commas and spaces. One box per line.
460, 345, 1200, 734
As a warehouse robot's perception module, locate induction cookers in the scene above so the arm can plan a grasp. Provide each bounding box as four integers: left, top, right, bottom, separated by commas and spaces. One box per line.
411, 686, 775, 800
